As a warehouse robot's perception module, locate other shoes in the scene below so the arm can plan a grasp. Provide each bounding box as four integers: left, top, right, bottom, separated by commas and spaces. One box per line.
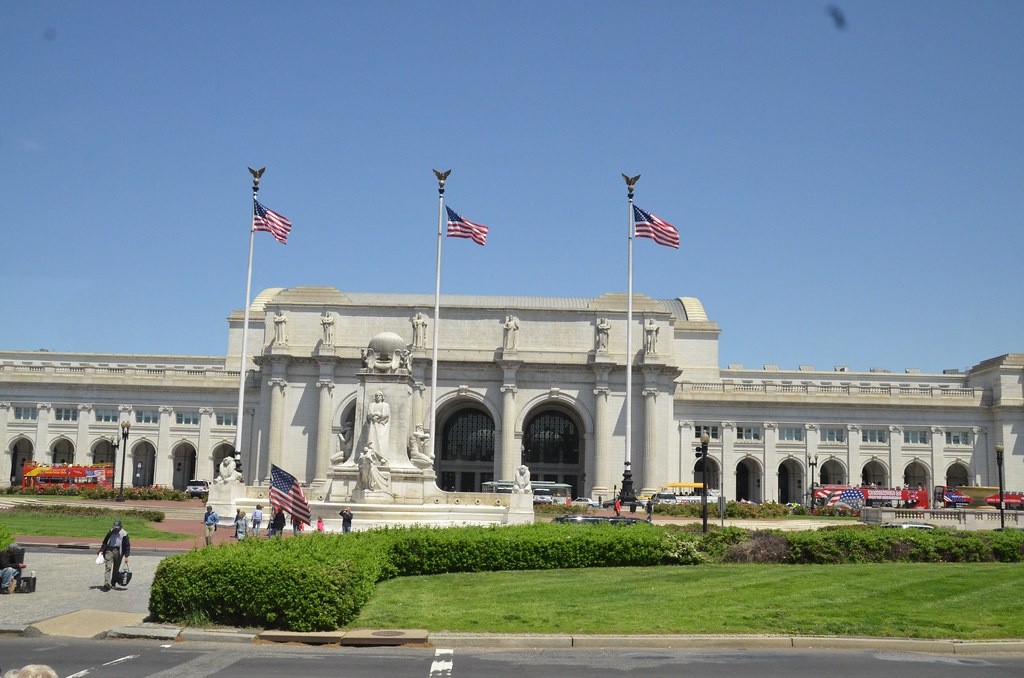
0, 587, 10, 594
111, 583, 115, 589
104, 584, 110, 590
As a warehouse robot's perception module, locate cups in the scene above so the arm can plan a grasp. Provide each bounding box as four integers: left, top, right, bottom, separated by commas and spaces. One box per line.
31, 570, 36, 578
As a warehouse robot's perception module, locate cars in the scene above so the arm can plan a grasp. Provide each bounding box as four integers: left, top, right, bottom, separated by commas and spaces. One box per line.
572, 496, 600, 509
646, 492, 678, 512
879, 521, 934, 532
602, 496, 619, 508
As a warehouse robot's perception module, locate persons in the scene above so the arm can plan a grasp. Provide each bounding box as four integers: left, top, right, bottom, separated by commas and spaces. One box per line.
503, 314, 519, 350
330, 422, 354, 465
265, 507, 285, 537
0, 543, 27, 594
317, 516, 324, 531
847, 482, 851, 488
251, 505, 264, 537
647, 500, 658, 521
203, 506, 219, 545
597, 317, 611, 350
408, 423, 435, 467
97, 520, 130, 590
273, 310, 288, 343
291, 514, 301, 533
904, 481, 909, 490
368, 391, 390, 455
412, 311, 428, 348
321, 312, 335, 345
615, 498, 621, 516
339, 507, 354, 534
646, 318, 660, 353
230, 508, 248, 542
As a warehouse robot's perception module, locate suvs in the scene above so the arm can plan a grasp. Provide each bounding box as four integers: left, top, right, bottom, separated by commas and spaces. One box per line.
185, 480, 212, 499
532, 489, 553, 506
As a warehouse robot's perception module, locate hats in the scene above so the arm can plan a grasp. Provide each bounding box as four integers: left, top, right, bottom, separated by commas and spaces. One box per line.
8, 543, 21, 551
113, 520, 121, 528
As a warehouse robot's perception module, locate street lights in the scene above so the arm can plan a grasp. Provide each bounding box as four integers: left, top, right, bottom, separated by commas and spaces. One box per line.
805, 452, 819, 513
995, 441, 1006, 529
117, 420, 132, 502
700, 428, 710, 533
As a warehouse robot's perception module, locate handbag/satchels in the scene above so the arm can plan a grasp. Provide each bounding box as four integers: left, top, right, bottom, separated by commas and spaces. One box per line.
15, 576, 36, 593
214, 524, 217, 531
96, 551, 105, 565
118, 563, 132, 585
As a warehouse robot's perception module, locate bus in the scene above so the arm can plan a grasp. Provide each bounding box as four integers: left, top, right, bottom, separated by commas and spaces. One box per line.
22, 460, 113, 493
811, 484, 929, 517
480, 479, 572, 504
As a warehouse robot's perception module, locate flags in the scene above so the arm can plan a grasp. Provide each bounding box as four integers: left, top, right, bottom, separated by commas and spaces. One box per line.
445, 204, 489, 247
632, 204, 679, 248
253, 197, 291, 245
269, 463, 312, 526
